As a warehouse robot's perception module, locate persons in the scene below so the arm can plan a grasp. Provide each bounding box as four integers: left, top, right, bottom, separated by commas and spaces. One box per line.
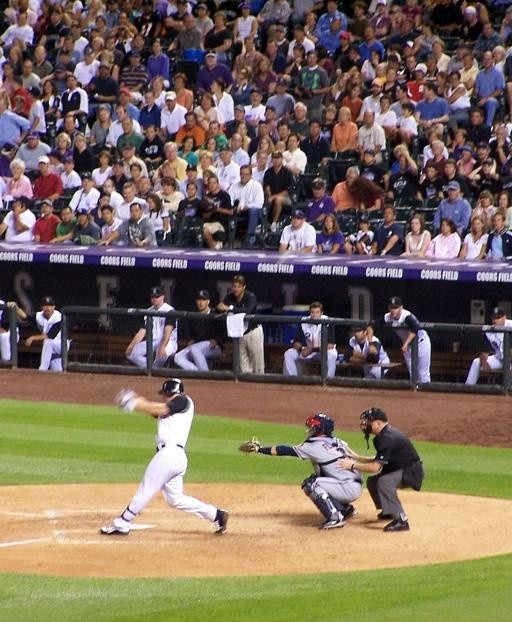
335, 408, 425, 532
2, 0, 511, 387
99, 378, 230, 536
240, 411, 364, 530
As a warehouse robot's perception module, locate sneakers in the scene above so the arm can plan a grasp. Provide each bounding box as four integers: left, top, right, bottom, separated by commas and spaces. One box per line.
100, 518, 130, 536
214, 509, 230, 534
383, 518, 409, 531
343, 504, 358, 519
320, 511, 346, 530
378, 509, 393, 519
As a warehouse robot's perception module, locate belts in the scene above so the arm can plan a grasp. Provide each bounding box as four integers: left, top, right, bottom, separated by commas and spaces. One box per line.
156, 444, 184, 452
243, 322, 261, 335
355, 479, 362, 484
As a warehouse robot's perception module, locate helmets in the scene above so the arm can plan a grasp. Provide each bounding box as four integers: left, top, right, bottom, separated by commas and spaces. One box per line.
359, 407, 388, 440
157, 378, 184, 397
232, 275, 247, 284
305, 413, 334, 441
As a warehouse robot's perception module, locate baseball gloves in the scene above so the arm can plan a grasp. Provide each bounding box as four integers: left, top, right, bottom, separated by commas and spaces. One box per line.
239, 436, 260, 453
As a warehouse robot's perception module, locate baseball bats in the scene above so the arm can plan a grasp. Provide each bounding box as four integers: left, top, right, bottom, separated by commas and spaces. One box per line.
86, 323, 102, 362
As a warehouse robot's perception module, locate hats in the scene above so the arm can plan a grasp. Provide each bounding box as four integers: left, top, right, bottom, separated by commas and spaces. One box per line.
376, 0, 386, 6
491, 306, 505, 319
388, 296, 402, 308
148, 286, 163, 298
185, 49, 324, 219
41, 297, 53, 306
358, 215, 369, 224
339, 32, 349, 39
363, 148, 374, 155
465, 6, 476, 15
195, 290, 209, 300
196, 2, 284, 33
13, 20, 176, 216
371, 78, 383, 87
446, 181, 460, 192
405, 40, 428, 75
477, 142, 493, 165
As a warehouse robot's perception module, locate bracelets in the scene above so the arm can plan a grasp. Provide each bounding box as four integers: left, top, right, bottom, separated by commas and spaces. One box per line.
351, 464, 356, 470
